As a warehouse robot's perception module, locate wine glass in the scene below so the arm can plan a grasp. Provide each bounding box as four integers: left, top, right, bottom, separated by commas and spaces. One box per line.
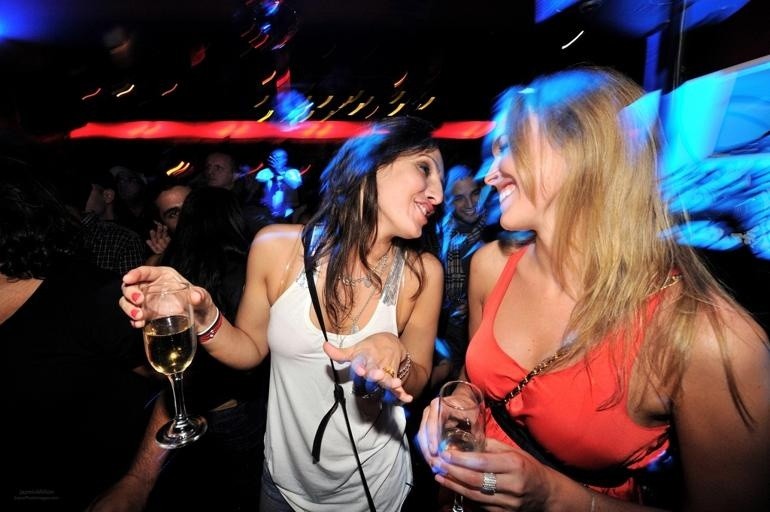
139, 280, 209, 452
436, 380, 487, 512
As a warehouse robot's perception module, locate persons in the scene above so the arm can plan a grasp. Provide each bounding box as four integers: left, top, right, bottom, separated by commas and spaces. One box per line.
1, 66, 769, 512
